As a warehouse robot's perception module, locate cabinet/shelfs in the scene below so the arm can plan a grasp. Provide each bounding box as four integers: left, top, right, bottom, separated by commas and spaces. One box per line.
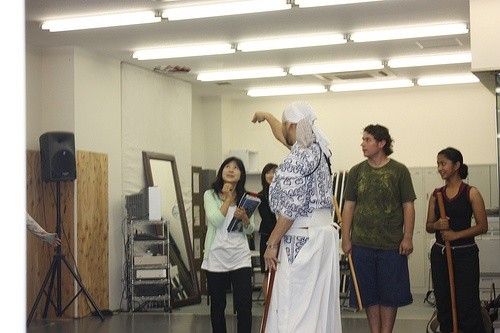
470, 0, 500, 95
127, 221, 173, 313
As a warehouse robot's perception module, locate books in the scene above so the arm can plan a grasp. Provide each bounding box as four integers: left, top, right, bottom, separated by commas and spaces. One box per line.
226, 192, 262, 233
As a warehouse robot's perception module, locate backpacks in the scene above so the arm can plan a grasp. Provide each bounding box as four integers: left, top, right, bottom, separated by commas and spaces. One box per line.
481, 306, 494, 333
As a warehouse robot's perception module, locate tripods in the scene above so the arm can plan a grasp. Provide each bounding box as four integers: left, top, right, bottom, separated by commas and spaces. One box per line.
26, 182, 105, 328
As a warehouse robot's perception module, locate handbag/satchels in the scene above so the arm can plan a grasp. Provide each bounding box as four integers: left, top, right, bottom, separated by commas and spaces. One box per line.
485, 282, 499, 321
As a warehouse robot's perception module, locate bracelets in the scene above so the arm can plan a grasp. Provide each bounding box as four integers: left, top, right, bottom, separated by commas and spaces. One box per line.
264, 240, 279, 249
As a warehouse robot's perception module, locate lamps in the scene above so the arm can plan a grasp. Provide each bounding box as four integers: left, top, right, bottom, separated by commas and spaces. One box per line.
39, 0, 481, 98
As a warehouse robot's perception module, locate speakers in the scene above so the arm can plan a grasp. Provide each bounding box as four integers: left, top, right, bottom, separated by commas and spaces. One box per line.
39, 131, 76, 182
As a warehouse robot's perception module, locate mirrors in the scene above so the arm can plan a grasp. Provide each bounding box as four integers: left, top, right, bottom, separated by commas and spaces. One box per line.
142, 151, 201, 308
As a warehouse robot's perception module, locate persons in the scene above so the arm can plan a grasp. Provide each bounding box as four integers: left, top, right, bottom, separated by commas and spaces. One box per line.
341, 124, 417, 332
256, 162, 279, 302
201, 156, 261, 333
27, 212, 62, 247
425, 147, 488, 332
249, 104, 342, 333
